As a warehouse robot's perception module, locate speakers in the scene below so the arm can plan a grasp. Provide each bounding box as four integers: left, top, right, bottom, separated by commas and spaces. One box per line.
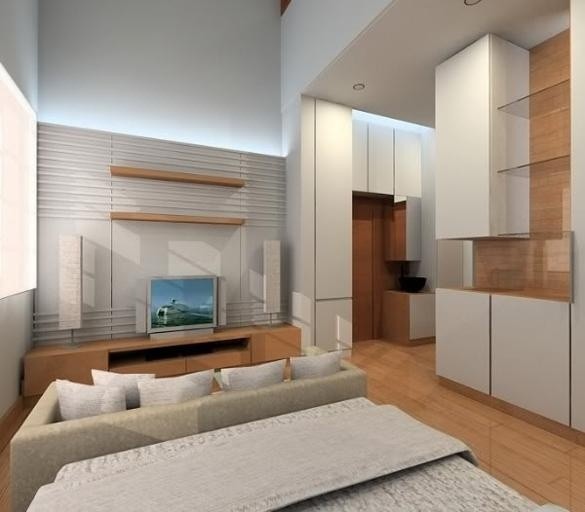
263, 239, 281, 314
59, 236, 83, 330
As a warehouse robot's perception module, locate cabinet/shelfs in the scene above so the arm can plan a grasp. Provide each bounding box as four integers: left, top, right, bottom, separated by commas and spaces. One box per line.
435, 288, 571, 427
353, 121, 422, 199
21, 322, 303, 396
383, 292, 435, 340
109, 164, 247, 225
434, 33, 530, 243
496, 77, 572, 238
384, 196, 421, 262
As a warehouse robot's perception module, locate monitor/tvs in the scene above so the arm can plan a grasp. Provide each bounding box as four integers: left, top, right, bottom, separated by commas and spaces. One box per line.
135, 275, 226, 342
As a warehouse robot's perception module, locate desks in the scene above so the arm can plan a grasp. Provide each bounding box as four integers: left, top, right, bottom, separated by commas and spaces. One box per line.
54, 397, 556, 511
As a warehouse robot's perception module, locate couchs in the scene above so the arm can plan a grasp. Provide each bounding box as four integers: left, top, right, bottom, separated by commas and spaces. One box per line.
6, 344, 369, 512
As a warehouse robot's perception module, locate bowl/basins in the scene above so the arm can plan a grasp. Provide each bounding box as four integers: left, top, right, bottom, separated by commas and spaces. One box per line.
398, 277, 426, 293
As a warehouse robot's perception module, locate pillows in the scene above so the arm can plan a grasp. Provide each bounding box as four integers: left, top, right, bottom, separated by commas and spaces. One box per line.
55, 347, 343, 419
